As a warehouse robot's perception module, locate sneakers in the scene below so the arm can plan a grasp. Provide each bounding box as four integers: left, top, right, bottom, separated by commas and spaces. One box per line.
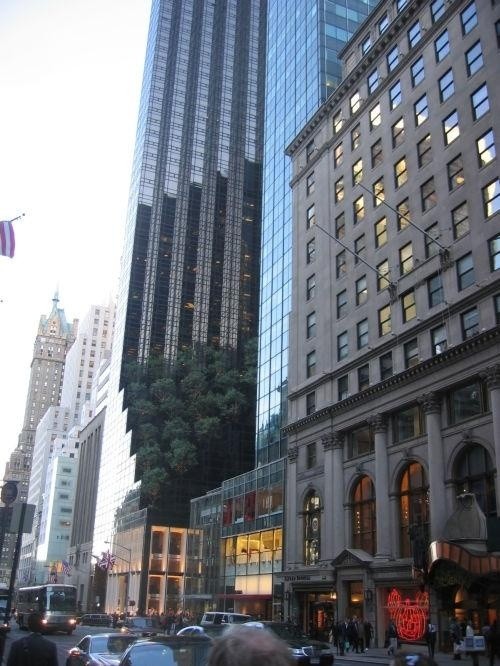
340, 648, 368, 656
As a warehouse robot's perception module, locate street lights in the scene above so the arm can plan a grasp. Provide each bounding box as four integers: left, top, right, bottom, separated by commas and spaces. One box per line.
103, 539, 131, 610
42, 564, 58, 583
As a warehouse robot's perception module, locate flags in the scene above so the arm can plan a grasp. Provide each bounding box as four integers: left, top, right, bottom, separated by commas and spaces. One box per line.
99, 552, 116, 570
0, 221, 15, 258
48, 566, 58, 584
61, 560, 72, 577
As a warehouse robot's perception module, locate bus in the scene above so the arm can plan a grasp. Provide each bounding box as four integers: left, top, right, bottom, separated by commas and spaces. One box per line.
0, 582, 13, 619
16, 582, 79, 635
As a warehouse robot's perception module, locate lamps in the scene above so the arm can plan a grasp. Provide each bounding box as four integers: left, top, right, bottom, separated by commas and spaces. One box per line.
328, 591, 337, 601
363, 589, 373, 602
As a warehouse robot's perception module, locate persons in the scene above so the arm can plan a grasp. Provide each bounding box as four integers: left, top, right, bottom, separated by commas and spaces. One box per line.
3, 607, 499, 666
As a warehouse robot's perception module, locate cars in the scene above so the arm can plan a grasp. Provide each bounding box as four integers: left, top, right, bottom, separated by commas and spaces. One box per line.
11, 606, 18, 620
61, 609, 334, 665
